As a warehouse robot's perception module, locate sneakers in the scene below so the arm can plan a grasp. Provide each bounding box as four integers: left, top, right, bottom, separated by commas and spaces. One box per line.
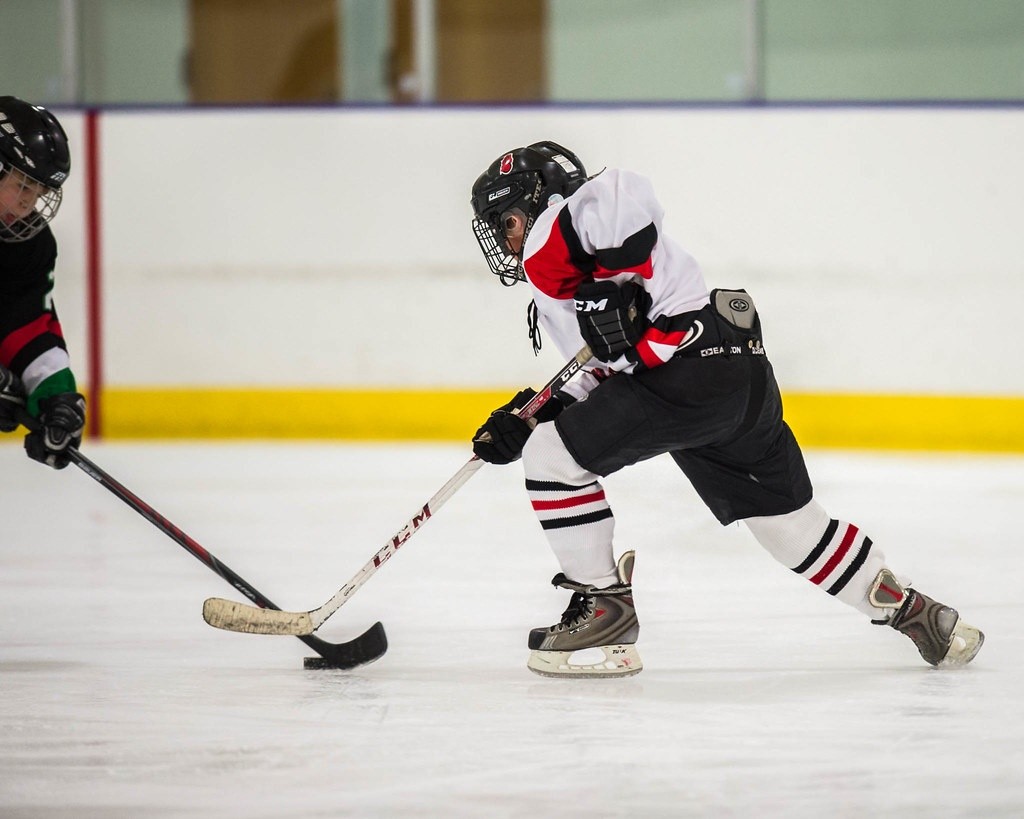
528, 550, 644, 679
868, 569, 985, 671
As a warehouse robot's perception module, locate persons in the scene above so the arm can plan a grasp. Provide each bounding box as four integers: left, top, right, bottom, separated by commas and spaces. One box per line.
472, 139, 986, 677
0, 93, 87, 470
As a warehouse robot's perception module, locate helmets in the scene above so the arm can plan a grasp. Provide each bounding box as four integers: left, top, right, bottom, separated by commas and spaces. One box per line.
471, 140, 587, 287
0, 95, 71, 242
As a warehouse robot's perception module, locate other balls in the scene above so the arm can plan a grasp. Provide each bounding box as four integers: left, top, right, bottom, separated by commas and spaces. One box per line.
303, 656, 333, 671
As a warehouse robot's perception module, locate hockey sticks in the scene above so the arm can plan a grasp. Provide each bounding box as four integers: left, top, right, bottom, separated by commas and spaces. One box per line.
199, 302, 638, 638
13, 407, 389, 673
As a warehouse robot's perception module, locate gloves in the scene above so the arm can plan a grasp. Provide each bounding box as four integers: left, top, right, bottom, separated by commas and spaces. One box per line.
0, 367, 23, 431
471, 387, 559, 466
574, 277, 652, 364
24, 392, 85, 469
